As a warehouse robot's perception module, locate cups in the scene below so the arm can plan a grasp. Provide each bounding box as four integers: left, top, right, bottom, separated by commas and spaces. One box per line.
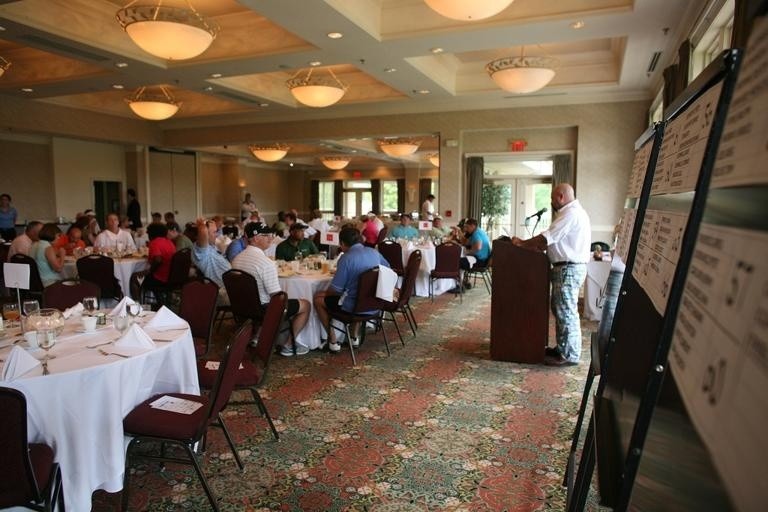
378, 231, 468, 254
59, 246, 147, 264
276, 252, 338, 273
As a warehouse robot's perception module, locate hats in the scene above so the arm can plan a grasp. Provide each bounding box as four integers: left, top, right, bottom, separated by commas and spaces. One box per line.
245, 222, 274, 238
289, 223, 308, 232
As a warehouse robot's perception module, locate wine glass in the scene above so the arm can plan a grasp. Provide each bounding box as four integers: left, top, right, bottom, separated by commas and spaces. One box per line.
25, 300, 39, 330
83, 297, 99, 323
3, 304, 26, 334
127, 301, 142, 329
114, 318, 125, 340
32, 326, 61, 364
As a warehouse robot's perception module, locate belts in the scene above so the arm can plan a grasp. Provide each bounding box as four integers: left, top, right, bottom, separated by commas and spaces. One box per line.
552, 261, 573, 267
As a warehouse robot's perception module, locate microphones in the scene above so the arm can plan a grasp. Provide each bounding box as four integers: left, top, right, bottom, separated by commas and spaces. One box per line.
527, 208, 548, 220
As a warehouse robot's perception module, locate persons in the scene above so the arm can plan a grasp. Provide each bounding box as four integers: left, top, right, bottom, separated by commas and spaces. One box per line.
511, 182, 591, 366
1, 190, 495, 356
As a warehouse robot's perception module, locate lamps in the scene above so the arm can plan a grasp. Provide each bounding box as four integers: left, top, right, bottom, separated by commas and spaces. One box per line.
247, 142, 290, 161
284, 66, 350, 107
319, 155, 351, 169
485, 44, 560, 95
121, 84, 183, 120
376, 138, 422, 158
115, 0, 223, 59
424, 152, 439, 167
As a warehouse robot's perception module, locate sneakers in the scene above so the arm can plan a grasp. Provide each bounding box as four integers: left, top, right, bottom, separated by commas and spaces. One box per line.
449, 286, 463, 292
319, 339, 341, 351
248, 335, 259, 347
464, 283, 471, 289
280, 345, 309, 356
545, 346, 570, 365
350, 336, 359, 348
